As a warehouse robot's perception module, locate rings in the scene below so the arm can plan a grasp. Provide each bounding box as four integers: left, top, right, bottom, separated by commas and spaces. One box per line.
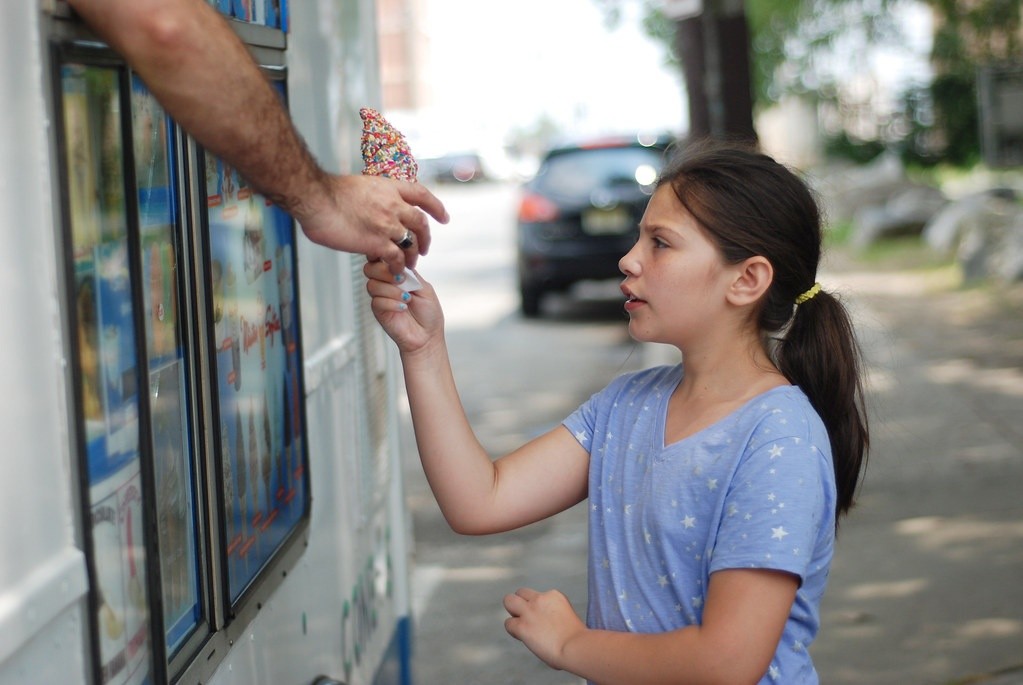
395, 228, 415, 250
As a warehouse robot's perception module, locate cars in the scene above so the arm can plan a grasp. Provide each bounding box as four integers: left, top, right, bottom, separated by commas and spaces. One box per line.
513, 129, 681, 318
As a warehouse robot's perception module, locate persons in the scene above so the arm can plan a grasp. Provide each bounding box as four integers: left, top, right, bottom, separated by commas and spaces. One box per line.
63, 1, 449, 276
364, 141, 869, 685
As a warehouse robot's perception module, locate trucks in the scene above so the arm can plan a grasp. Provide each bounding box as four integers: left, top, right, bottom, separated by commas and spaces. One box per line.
1, 0, 421, 683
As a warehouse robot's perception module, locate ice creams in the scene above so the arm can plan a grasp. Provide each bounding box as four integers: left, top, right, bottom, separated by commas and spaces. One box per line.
357, 107, 417, 182
